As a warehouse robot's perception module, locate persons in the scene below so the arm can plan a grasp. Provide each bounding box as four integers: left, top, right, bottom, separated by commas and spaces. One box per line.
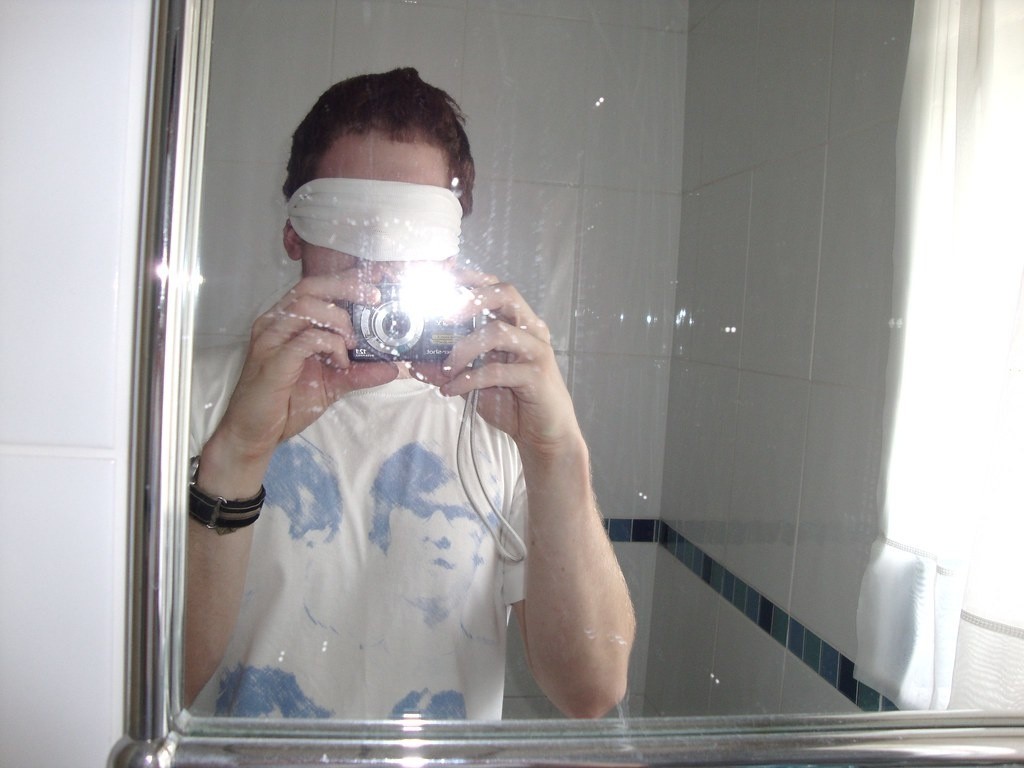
180, 66, 635, 723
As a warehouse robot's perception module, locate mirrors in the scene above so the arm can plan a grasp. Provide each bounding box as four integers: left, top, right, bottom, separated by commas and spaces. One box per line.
106, 0, 1024, 768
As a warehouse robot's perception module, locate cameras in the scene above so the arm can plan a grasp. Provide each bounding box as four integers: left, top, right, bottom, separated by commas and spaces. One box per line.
343, 281, 487, 364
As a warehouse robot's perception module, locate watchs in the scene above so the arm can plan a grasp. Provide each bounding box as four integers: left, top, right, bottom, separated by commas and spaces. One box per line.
188, 456, 267, 533
